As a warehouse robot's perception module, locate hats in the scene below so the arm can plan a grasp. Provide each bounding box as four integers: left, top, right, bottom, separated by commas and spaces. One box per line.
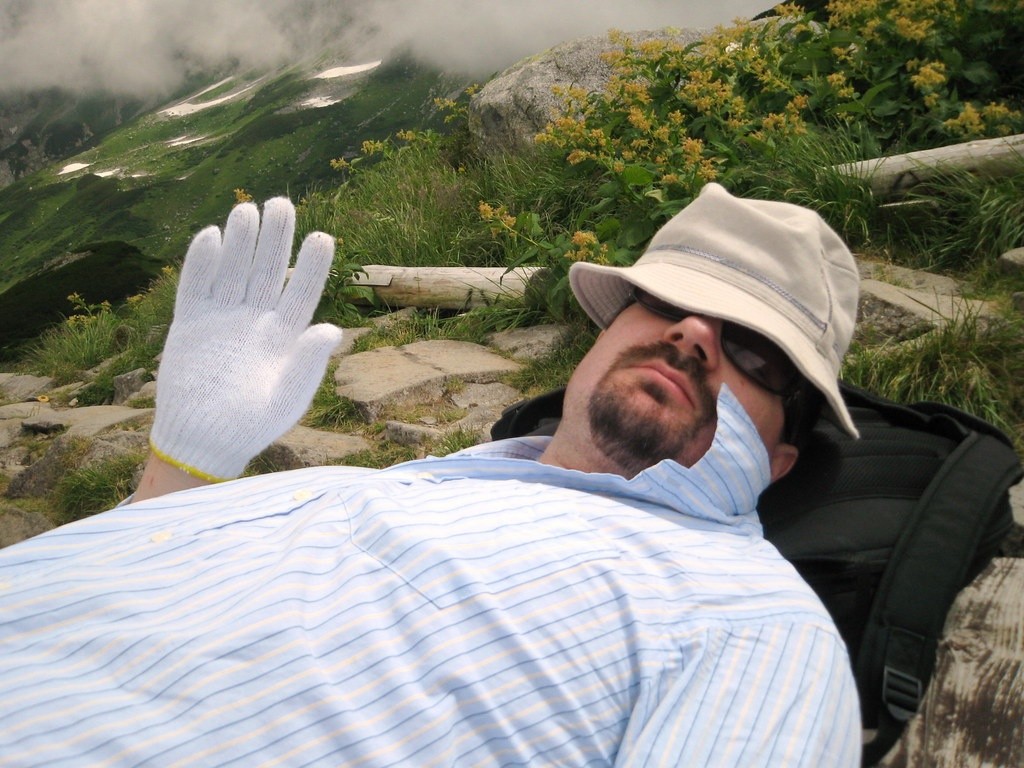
569, 183, 861, 440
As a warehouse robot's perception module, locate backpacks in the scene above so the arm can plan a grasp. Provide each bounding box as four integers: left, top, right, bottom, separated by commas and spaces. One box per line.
490, 361, 1024, 768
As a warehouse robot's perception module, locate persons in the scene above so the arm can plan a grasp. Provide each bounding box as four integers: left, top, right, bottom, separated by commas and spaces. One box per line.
0, 183, 862, 768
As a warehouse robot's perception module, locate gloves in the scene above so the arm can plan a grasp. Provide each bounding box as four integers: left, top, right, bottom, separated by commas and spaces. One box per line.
149, 196, 343, 481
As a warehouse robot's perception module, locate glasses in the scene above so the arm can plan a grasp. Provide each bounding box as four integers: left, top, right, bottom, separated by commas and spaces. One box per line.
621, 285, 804, 409
